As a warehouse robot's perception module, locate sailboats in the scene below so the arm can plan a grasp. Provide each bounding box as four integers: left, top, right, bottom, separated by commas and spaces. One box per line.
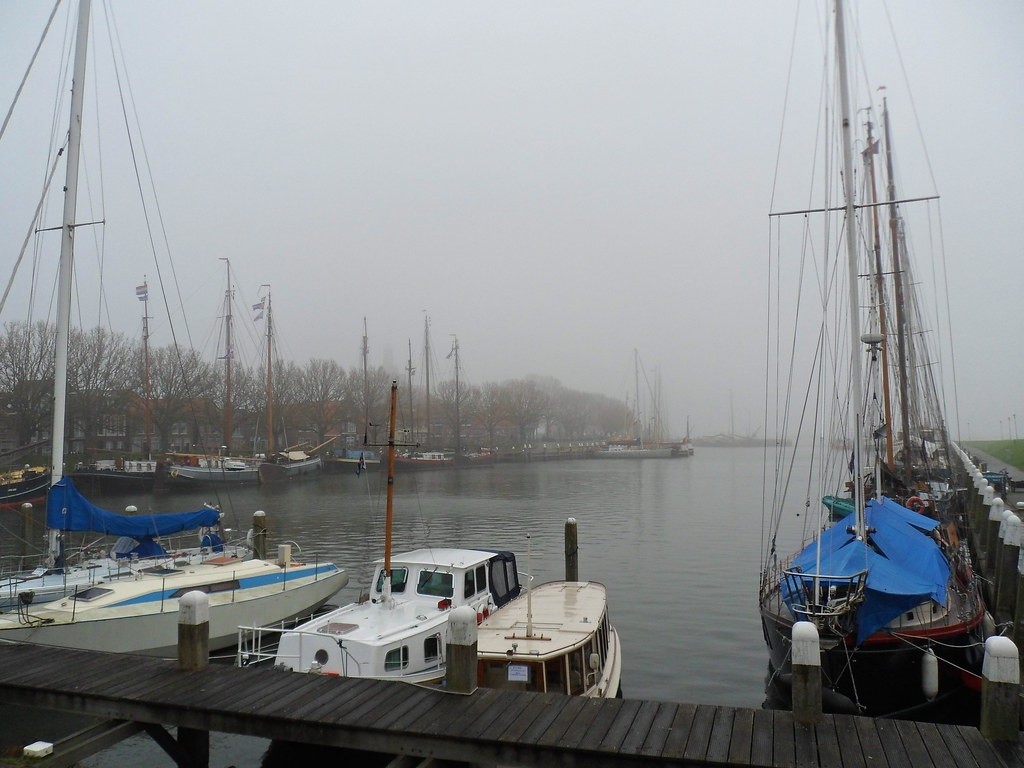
0, 2, 262, 611
66, 258, 696, 497
760, 0, 992, 722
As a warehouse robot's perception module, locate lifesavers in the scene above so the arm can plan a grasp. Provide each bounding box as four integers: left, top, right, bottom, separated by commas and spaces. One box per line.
905, 496, 926, 515
476, 604, 490, 625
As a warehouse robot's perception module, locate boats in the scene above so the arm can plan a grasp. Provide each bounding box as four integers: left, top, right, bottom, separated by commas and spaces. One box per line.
447, 517, 622, 698
0, 464, 51, 510
1, 540, 349, 660
275, 381, 524, 686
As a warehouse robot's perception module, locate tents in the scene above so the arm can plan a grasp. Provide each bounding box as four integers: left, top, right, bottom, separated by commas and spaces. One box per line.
110, 535, 170, 561
200, 534, 224, 554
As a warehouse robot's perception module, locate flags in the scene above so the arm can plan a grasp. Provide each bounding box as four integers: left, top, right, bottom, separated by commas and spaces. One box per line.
355, 453, 367, 476
253, 296, 269, 321
